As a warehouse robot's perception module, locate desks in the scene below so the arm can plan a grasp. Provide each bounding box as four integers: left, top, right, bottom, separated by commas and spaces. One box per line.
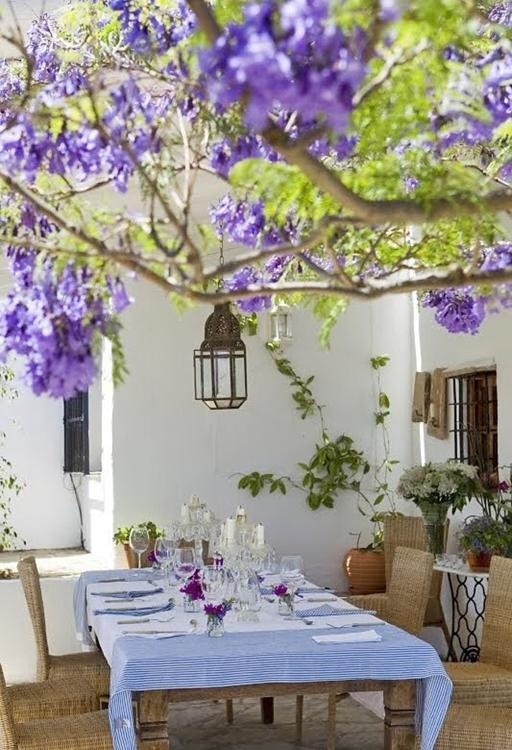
433, 558, 490, 662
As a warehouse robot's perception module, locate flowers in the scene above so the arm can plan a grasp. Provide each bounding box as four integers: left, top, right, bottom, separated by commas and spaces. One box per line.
454, 516, 512, 555
178, 568, 206, 602
271, 584, 295, 606
200, 595, 235, 620
147, 550, 159, 562
394, 460, 481, 557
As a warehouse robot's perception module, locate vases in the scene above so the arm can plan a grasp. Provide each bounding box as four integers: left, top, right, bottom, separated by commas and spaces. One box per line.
467, 549, 493, 572
151, 560, 161, 569
419, 501, 449, 561
183, 594, 195, 612
208, 615, 224, 637
277, 597, 293, 616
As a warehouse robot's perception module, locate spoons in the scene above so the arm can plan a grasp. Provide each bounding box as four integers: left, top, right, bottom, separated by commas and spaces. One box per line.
292, 598, 337, 603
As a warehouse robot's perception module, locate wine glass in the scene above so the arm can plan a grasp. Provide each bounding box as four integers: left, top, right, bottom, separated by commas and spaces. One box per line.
155, 537, 197, 599
202, 564, 224, 620
278, 554, 305, 623
125, 525, 150, 578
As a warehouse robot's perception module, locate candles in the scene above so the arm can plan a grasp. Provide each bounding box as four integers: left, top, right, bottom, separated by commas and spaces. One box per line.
202, 510, 211, 525
254, 522, 264, 545
180, 503, 191, 524
226, 516, 236, 541
236, 506, 244, 516
191, 495, 198, 506
221, 522, 225, 538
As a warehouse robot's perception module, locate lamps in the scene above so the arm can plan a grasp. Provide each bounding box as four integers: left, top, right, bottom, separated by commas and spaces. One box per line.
192, 219, 248, 410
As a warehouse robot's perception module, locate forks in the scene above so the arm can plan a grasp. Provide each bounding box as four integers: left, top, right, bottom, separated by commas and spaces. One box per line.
117, 616, 177, 624
325, 621, 385, 629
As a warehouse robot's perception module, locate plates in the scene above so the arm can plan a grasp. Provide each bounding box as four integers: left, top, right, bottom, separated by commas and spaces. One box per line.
93, 599, 176, 613
90, 580, 162, 597
298, 593, 337, 602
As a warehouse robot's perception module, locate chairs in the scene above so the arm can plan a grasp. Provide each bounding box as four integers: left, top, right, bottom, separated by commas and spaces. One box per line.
124, 538, 208, 571
342, 513, 457, 662
375, 546, 434, 637
14, 554, 139, 729
0, 665, 140, 749
415, 554, 512, 750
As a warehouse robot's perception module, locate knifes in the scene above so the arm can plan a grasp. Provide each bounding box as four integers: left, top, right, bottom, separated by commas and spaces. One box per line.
120, 628, 191, 637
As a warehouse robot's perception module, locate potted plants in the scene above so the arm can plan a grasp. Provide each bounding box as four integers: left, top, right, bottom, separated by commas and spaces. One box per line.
228, 345, 401, 594
113, 522, 165, 569
